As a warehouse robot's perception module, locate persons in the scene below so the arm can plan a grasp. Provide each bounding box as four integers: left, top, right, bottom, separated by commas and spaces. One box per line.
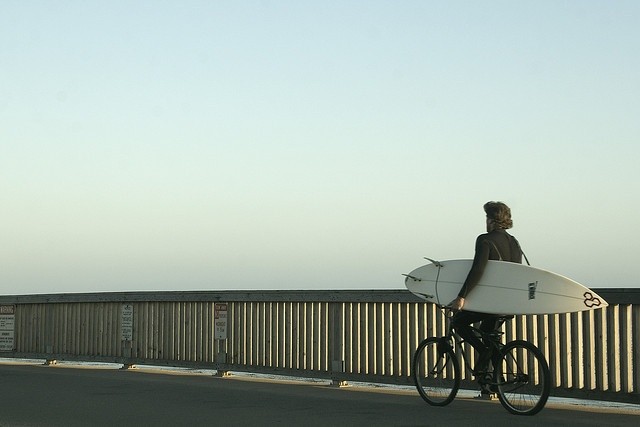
447, 201, 521, 394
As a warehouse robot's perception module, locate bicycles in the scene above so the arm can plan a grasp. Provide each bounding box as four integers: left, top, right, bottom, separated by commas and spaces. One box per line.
413, 304, 551, 416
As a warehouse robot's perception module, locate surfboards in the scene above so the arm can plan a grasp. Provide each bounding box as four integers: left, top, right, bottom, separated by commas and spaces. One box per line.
404, 259, 610, 315
